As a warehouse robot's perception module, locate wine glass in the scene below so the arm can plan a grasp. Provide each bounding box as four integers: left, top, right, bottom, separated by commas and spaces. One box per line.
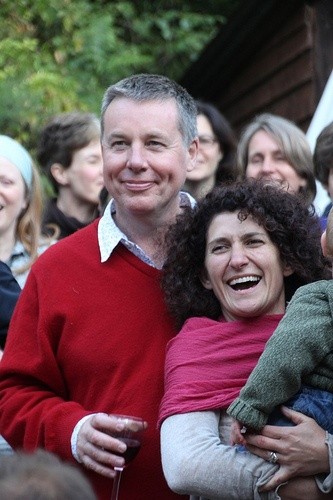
108, 413, 144, 500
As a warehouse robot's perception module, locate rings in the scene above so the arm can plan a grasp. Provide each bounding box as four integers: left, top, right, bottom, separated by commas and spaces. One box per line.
268, 451, 278, 464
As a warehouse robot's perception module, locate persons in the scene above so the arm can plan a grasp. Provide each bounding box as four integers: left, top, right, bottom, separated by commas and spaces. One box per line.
238, 113, 317, 207
180, 103, 239, 202
157, 175, 333, 500
0, 134, 61, 359
33, 113, 106, 241
0, 74, 200, 500
226, 206, 333, 450
312, 120, 333, 253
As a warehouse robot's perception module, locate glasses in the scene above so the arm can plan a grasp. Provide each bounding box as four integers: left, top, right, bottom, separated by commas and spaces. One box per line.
197, 135, 220, 149
